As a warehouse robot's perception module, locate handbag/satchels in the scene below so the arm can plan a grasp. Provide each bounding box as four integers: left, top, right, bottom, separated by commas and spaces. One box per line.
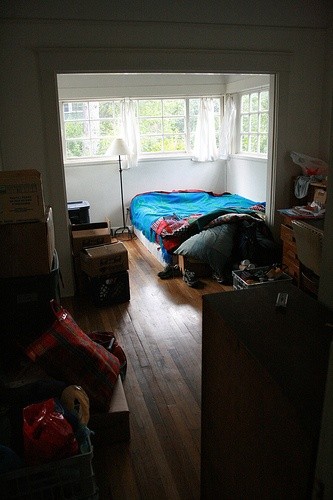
290, 151, 329, 176
18, 299, 121, 414
88, 330, 127, 382
19, 398, 80, 468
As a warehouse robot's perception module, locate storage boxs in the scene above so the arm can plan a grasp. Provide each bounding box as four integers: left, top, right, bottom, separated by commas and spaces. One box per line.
0, 266, 63, 336
0, 168, 46, 223
0, 205, 55, 280
85, 270, 130, 307
90, 372, 132, 447
0, 421, 99, 500
71, 227, 111, 253
231, 264, 292, 290
78, 241, 130, 277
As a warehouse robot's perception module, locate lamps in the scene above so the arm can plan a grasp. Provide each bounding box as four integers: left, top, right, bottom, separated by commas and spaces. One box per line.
105, 136, 135, 240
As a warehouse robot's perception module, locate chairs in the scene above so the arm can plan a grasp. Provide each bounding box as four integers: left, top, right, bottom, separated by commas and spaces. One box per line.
289, 217, 323, 295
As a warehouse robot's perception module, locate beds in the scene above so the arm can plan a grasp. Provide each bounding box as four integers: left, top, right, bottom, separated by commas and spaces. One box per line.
127, 189, 266, 271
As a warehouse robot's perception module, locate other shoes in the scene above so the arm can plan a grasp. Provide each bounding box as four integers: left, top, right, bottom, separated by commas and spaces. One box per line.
157, 264, 181, 280
183, 269, 200, 287
211, 270, 227, 284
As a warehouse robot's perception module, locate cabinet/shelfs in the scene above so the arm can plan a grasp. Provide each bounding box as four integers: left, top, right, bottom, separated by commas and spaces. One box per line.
197, 281, 333, 500
279, 207, 324, 295
